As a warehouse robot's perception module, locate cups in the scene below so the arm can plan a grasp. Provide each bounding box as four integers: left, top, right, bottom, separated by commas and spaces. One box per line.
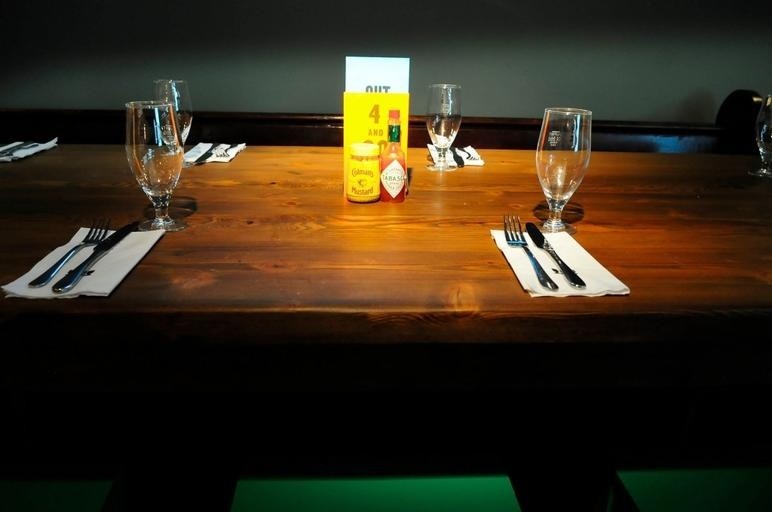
154, 78, 193, 153
347, 142, 381, 203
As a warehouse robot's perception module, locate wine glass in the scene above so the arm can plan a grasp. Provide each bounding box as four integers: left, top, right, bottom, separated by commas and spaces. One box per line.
426, 83, 463, 172
746, 94, 772, 179
534, 107, 594, 236
125, 99, 189, 232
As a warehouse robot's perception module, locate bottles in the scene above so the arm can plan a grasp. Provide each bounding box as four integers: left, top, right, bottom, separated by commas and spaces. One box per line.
379, 108, 408, 203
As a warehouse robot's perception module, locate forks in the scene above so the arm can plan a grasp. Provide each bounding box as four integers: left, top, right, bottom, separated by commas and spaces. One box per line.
26, 217, 113, 288
216, 143, 238, 157
504, 212, 558, 292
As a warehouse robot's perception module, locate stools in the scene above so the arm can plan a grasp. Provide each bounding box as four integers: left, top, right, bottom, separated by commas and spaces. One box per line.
0, 478, 112, 512
604, 466, 771, 512
230, 474, 522, 512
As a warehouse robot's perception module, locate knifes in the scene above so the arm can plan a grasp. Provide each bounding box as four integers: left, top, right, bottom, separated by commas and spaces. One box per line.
193, 142, 220, 164
525, 222, 586, 289
52, 219, 140, 293
